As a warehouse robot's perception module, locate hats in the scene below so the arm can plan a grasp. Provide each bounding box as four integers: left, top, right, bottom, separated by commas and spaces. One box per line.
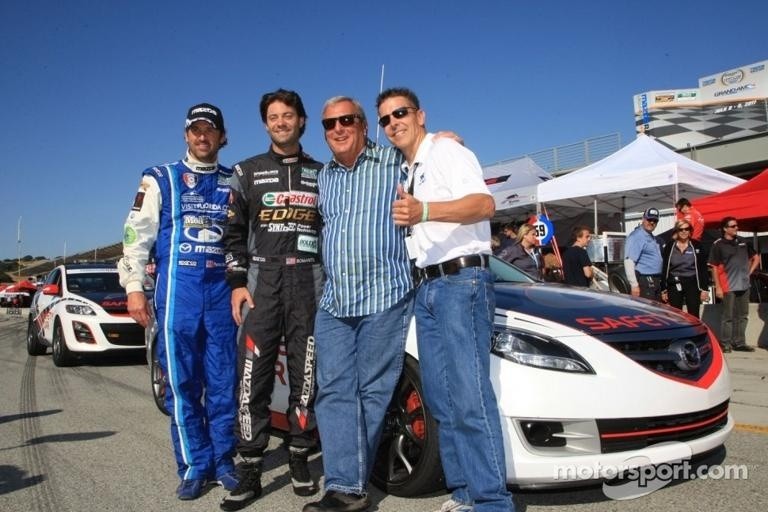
185, 103, 222, 130
643, 207, 658, 221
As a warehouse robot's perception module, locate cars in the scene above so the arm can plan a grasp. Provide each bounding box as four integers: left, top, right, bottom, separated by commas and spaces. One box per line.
150, 251, 735, 499
25, 262, 148, 366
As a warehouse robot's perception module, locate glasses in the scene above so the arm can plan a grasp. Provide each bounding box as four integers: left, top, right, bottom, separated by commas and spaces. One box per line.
725, 225, 737, 228
678, 205, 682, 212
378, 107, 418, 127
646, 218, 657, 223
321, 114, 361, 129
504, 230, 509, 233
677, 227, 690, 231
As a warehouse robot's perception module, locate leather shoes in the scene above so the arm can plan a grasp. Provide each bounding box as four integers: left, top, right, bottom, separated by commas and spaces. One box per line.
734, 345, 754, 352
721, 345, 731, 353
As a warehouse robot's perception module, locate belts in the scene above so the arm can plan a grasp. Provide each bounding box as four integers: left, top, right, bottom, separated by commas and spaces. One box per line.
413, 252, 488, 284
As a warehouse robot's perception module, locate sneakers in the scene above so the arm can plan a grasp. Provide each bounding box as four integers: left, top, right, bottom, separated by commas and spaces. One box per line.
302, 489, 370, 512
178, 467, 209, 500
435, 500, 474, 512
206, 466, 246, 492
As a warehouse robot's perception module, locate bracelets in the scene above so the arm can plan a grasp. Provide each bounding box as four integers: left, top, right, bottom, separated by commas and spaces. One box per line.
420, 201, 428, 223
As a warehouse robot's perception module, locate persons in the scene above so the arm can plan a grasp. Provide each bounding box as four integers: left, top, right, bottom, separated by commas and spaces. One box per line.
375, 86, 516, 511
496, 223, 543, 281
501, 224, 518, 250
671, 198, 704, 242
623, 208, 664, 301
660, 220, 708, 319
562, 225, 593, 288
706, 217, 761, 352
300, 94, 466, 512
223, 88, 327, 511
116, 104, 243, 501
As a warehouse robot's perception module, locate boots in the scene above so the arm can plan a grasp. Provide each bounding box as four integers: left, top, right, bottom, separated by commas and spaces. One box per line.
219, 453, 263, 512
287, 447, 315, 497
